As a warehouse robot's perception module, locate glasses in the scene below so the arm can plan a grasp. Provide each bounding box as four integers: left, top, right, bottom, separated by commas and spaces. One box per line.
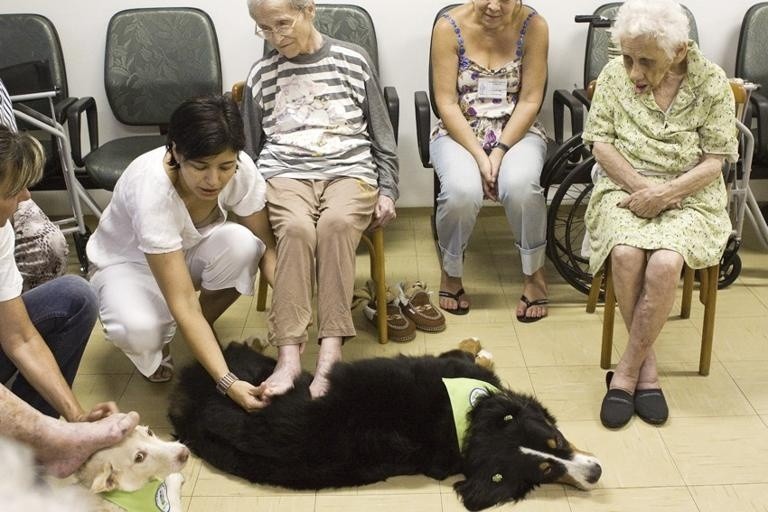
254, 2, 305, 40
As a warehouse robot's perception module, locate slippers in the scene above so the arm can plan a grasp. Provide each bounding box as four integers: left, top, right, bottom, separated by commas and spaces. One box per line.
518, 294, 549, 323
439, 288, 469, 315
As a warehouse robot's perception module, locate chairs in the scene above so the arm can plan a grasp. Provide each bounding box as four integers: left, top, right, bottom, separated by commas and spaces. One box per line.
70, 7, 224, 262
239, 4, 390, 347
0, 11, 68, 249
577, 7, 698, 154
734, 2, 767, 259
591, 83, 748, 378
410, 3, 554, 248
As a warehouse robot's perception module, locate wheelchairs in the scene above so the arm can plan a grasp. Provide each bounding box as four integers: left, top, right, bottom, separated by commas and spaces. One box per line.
539, 14, 767, 300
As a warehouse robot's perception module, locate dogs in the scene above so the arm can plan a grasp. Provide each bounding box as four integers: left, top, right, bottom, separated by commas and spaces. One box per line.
0, 412, 191, 512
166, 334, 603, 512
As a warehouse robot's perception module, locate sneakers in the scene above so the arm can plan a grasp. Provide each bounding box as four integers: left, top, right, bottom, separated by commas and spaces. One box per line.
599, 371, 634, 428
634, 389, 668, 425
396, 280, 445, 331
363, 297, 416, 341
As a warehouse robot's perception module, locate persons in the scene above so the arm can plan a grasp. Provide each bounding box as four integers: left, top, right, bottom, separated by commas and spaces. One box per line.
579, 0, 741, 427
85, 94, 278, 418
241, 0, 396, 401
0, 80, 139, 479
428, 1, 550, 324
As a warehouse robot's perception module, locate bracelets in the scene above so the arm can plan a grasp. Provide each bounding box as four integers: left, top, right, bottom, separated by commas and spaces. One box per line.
491, 142, 509, 152
214, 367, 239, 397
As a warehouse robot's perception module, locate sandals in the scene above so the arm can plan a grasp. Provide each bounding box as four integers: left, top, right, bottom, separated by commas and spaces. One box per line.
145, 352, 174, 382
